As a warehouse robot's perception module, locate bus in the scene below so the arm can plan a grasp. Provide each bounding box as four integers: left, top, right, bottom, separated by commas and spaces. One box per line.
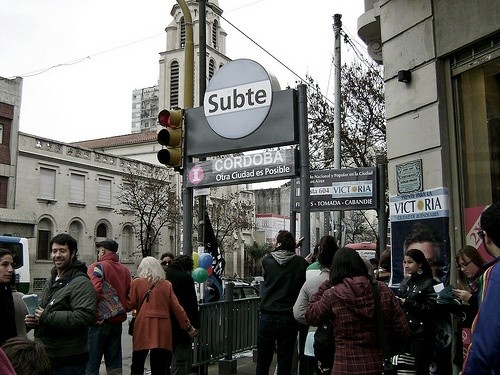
0, 235, 31, 294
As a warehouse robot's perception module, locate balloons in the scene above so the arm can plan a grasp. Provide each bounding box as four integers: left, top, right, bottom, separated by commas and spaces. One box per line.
193, 251, 214, 284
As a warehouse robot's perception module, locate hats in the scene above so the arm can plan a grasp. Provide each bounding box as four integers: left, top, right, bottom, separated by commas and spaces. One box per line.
95, 240, 118, 252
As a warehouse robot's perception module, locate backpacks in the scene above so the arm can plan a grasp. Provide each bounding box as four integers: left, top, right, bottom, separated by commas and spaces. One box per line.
314, 311, 335, 369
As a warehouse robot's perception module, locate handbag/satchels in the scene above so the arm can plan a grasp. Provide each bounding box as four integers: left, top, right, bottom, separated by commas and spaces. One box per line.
128, 317, 136, 336
381, 337, 417, 375
96, 281, 124, 321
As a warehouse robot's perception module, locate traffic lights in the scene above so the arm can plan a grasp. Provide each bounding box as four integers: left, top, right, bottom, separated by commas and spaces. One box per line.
156, 108, 184, 168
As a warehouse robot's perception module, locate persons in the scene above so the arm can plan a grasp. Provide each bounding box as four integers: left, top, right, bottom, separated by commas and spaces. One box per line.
127, 256, 197, 375
164, 255, 199, 375
396, 250, 437, 375
293, 235, 338, 375
257, 231, 309, 375
459, 204, 500, 375
161, 253, 174, 267
24, 233, 98, 375
446, 244, 485, 369
87, 241, 132, 375
365, 250, 390, 282
403, 225, 446, 282
195, 272, 221, 375
305, 248, 410, 375
0, 337, 50, 375
0, 249, 34, 343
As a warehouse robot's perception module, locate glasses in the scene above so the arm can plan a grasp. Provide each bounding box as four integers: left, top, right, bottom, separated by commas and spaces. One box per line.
478, 231, 485, 238
161, 260, 172, 266
456, 260, 473, 270
